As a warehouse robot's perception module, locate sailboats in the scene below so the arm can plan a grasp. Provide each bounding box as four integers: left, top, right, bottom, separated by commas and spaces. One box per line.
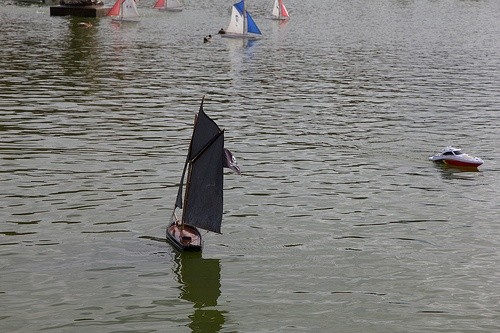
151, 0, 183, 12
272, 0, 289, 20
108, 0, 141, 23
165, 95, 240, 251
221, 0, 262, 39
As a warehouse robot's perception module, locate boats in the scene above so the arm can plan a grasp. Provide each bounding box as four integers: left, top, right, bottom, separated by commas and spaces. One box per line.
429, 146, 483, 167
